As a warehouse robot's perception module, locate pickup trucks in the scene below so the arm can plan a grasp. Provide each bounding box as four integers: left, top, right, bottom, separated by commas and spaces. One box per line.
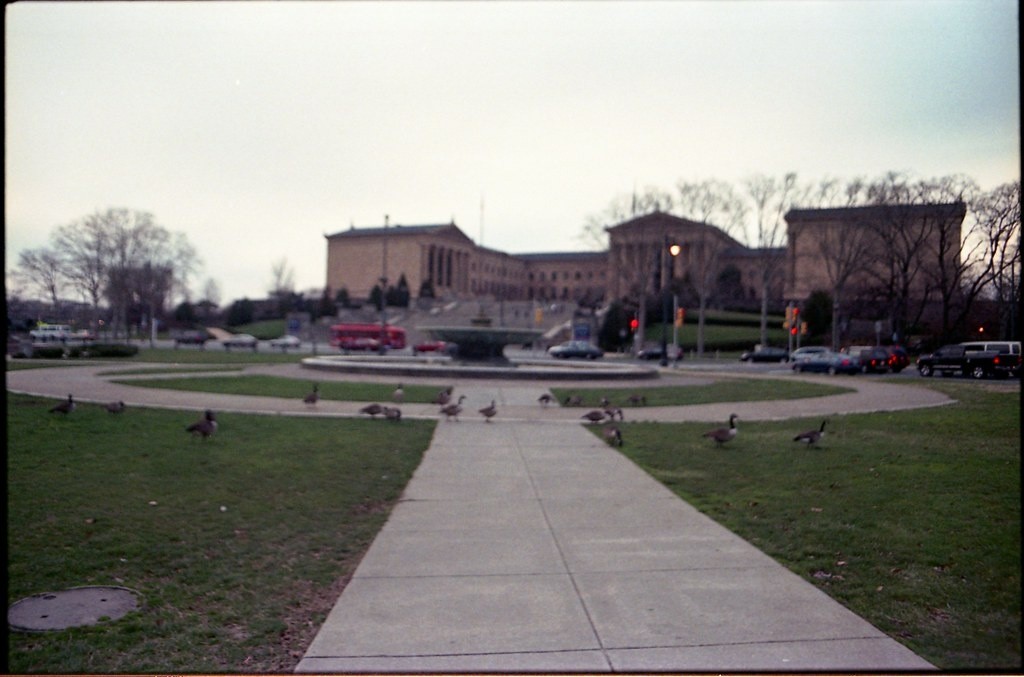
30, 324, 67, 342
915, 345, 1021, 380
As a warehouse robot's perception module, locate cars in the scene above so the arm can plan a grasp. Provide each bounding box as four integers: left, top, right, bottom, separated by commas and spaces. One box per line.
223, 334, 256, 349
271, 334, 301, 350
791, 352, 859, 375
741, 348, 790, 364
638, 348, 684, 362
413, 337, 446, 353
842, 346, 892, 375
176, 331, 207, 344
548, 341, 603, 360
790, 346, 830, 361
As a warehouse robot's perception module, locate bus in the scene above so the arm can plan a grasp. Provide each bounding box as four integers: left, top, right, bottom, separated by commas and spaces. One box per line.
329, 324, 406, 353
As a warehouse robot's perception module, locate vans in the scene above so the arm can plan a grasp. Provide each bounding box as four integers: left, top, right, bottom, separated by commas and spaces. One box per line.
960, 341, 1021, 357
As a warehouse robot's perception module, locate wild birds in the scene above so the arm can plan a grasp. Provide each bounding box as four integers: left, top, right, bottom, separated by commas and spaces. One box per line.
626, 395, 643, 406
537, 394, 554, 408
359, 404, 381, 420
383, 406, 401, 422
599, 394, 609, 407
793, 419, 828, 447
48, 395, 75, 416
581, 412, 605, 425
302, 386, 318, 403
432, 386, 453, 404
599, 427, 623, 447
391, 383, 403, 403
564, 396, 582, 406
701, 414, 738, 448
604, 408, 624, 421
98, 400, 124, 415
185, 410, 217, 438
480, 400, 497, 422
437, 395, 466, 421
